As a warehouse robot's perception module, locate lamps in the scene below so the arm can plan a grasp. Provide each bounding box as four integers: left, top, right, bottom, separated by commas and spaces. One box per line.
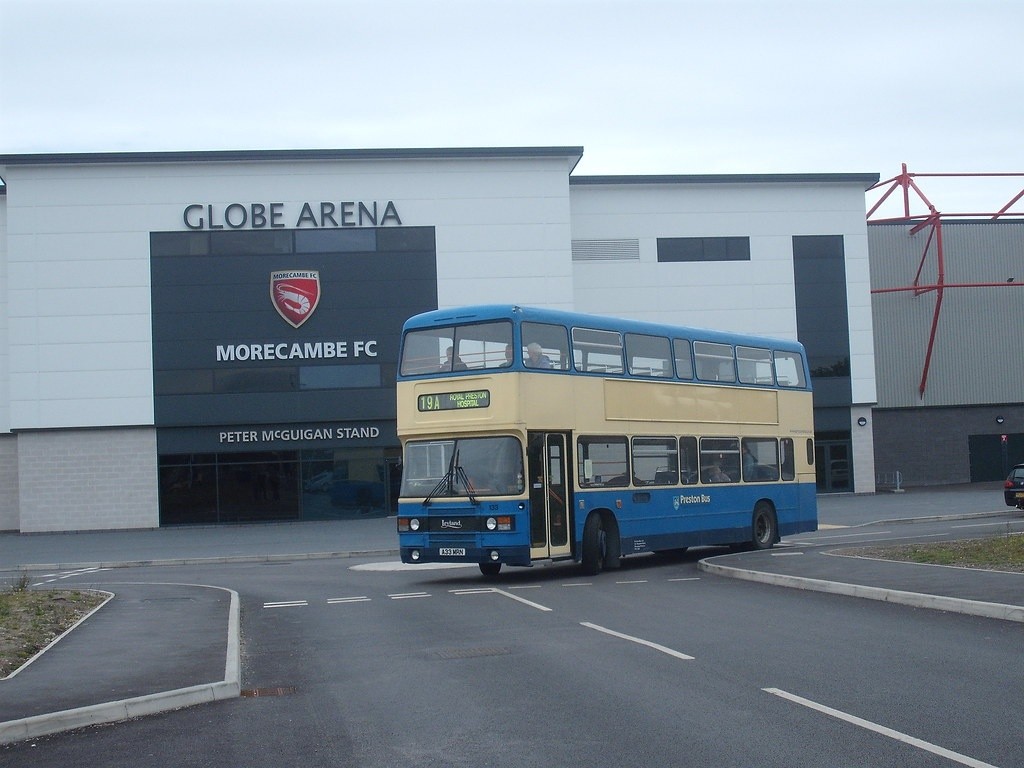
858, 417, 866, 426
996, 415, 1004, 423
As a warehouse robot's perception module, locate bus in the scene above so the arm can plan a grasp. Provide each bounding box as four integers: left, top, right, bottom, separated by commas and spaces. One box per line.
396, 303, 819, 576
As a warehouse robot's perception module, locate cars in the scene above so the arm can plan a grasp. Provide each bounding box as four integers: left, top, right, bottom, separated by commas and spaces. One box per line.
1003, 464, 1024, 510
306, 471, 336, 492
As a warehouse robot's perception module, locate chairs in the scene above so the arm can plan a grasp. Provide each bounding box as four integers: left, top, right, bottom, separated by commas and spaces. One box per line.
523, 359, 789, 387
644, 466, 777, 486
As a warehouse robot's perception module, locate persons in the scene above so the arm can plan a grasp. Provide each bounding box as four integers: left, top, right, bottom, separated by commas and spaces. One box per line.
526, 342, 550, 369
440, 347, 469, 372
706, 461, 731, 483
499, 344, 514, 368
